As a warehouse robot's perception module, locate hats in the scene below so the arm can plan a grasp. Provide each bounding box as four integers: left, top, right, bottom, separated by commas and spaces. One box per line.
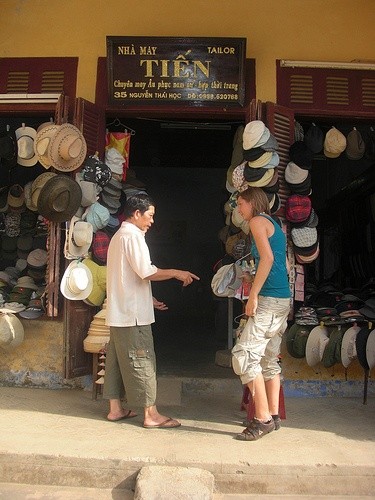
209, 120, 375, 372
0, 121, 126, 354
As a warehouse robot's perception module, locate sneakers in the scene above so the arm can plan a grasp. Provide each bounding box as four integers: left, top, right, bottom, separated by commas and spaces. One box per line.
235, 417, 276, 441
243, 414, 282, 430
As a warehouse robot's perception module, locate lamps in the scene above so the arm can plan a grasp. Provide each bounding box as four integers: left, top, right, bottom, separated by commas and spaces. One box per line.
160, 122, 231, 130
0, 94, 64, 103
279, 60, 375, 71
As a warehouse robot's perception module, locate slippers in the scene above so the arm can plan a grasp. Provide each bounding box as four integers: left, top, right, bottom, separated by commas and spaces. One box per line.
109, 410, 139, 421
143, 417, 181, 428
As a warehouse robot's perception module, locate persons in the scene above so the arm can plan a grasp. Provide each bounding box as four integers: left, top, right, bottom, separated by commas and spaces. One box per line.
102, 194, 201, 429
231, 188, 291, 440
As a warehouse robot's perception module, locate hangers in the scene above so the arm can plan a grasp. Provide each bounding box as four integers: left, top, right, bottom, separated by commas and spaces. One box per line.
106, 118, 135, 135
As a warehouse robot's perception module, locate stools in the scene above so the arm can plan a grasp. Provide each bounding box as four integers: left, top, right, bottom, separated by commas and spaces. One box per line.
240, 384, 285, 420
